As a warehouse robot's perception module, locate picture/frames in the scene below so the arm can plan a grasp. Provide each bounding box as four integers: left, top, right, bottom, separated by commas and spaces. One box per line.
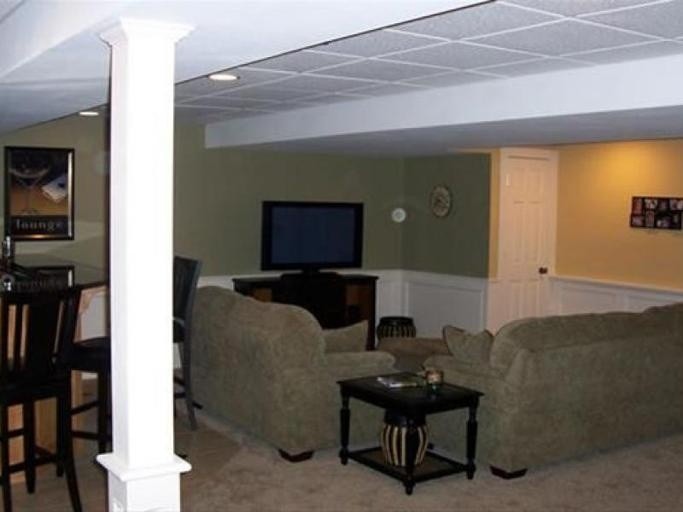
629, 194, 682, 231
2, 144, 77, 241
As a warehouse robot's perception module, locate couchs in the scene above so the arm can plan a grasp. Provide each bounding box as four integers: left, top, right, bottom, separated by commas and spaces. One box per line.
191, 289, 399, 464
415, 297, 683, 479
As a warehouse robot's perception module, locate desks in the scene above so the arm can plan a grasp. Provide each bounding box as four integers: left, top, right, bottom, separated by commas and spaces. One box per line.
0, 250, 110, 477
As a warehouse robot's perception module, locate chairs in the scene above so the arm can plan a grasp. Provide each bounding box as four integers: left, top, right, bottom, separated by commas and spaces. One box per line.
73, 245, 201, 463
1, 279, 88, 510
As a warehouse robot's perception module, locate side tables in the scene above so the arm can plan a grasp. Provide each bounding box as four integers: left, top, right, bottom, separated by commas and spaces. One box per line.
333, 372, 486, 496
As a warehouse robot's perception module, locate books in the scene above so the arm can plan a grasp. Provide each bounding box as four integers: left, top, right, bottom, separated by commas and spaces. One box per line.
376, 372, 428, 389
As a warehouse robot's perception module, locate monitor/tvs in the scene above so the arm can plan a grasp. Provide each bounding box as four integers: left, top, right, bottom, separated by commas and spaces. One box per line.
261, 201, 364, 279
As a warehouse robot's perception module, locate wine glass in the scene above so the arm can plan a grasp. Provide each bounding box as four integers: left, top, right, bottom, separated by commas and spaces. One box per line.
10, 165, 48, 215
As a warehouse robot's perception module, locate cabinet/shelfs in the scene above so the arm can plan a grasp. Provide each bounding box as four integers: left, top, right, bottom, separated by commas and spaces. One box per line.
231, 273, 382, 353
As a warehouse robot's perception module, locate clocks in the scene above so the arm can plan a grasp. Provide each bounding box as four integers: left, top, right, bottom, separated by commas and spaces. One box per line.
429, 183, 454, 220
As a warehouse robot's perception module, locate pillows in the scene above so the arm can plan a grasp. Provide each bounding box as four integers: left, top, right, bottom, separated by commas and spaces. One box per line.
441, 322, 496, 367
319, 317, 369, 355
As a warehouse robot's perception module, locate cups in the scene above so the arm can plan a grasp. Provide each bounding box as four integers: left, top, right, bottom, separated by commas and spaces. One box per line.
425, 369, 444, 395
